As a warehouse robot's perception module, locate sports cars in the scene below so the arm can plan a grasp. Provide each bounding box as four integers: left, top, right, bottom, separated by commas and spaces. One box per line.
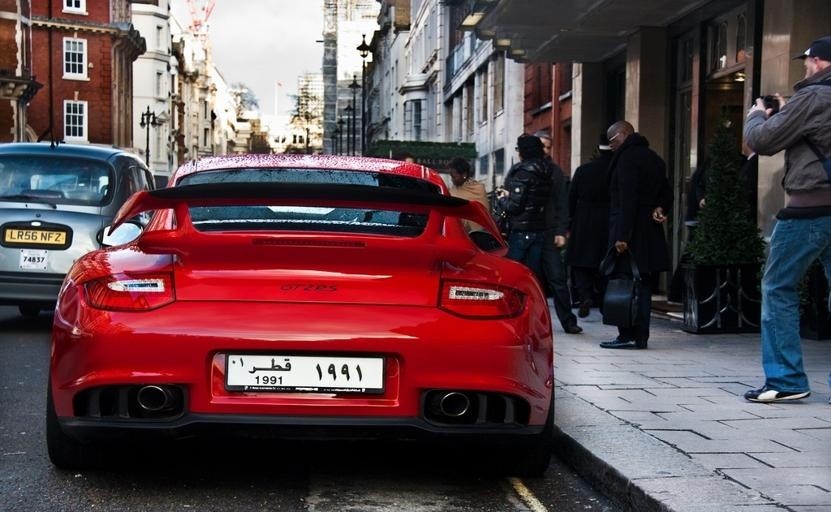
44, 152, 558, 488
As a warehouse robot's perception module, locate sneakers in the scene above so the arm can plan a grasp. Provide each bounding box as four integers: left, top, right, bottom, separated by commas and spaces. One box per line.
744, 384, 809, 403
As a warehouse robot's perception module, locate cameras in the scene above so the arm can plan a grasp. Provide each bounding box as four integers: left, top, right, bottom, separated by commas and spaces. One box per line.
759, 95, 779, 116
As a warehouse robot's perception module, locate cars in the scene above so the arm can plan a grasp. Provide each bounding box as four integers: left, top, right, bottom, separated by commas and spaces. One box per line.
1, 141, 158, 320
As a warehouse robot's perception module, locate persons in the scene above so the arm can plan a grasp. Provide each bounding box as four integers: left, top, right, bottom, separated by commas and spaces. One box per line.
403, 154, 417, 164
564, 132, 616, 319
599, 120, 674, 348
746, 35, 831, 403
738, 135, 761, 222
447, 156, 493, 231
496, 134, 552, 274
504, 131, 587, 333
686, 163, 709, 246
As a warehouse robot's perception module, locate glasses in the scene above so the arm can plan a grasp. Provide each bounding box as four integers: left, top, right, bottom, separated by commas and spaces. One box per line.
608, 133, 619, 143
515, 147, 519, 152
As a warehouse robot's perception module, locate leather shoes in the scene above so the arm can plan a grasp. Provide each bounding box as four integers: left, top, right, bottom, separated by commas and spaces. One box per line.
564, 324, 582, 334
577, 306, 589, 318
599, 338, 638, 349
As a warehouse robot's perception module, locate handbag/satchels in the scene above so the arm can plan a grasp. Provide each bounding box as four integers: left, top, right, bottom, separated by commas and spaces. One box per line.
601, 277, 640, 328
490, 201, 510, 236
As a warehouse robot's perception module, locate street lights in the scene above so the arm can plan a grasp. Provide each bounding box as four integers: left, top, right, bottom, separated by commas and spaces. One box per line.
139, 103, 160, 167
330, 34, 374, 155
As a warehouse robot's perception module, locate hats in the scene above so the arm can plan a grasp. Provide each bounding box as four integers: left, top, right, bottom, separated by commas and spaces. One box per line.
596, 135, 613, 151
792, 36, 830, 62
